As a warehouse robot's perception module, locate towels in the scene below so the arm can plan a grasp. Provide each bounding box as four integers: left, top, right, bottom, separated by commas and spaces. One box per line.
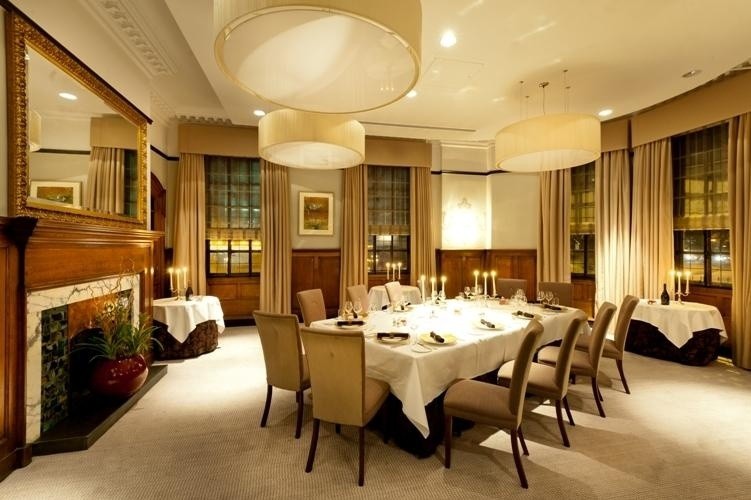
336, 303, 561, 342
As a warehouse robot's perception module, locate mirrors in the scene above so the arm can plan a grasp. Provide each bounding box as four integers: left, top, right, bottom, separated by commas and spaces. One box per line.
5, 12, 148, 230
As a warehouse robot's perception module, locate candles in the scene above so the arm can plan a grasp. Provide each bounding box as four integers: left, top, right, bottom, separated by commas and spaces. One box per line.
474, 270, 496, 295
670, 270, 690, 294
421, 275, 445, 299
386, 262, 401, 279
169, 267, 187, 290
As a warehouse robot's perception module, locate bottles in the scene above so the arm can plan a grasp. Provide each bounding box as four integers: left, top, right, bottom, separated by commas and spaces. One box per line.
661, 282, 671, 305
186, 279, 195, 301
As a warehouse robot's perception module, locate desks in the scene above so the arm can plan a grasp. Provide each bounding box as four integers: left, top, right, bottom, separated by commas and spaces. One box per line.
367, 285, 421, 312
624, 299, 729, 365
152, 296, 226, 360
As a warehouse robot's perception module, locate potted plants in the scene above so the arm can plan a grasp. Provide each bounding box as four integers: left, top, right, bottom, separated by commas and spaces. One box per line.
75, 256, 164, 398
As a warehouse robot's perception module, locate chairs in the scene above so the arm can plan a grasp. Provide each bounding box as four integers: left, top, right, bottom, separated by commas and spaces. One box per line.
497, 314, 588, 447
297, 289, 327, 326
538, 302, 617, 417
443, 321, 544, 487
252, 309, 389, 487
572, 295, 640, 394
385, 281, 404, 307
345, 284, 369, 312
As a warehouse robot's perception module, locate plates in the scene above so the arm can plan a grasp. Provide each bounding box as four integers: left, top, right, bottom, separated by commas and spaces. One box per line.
419, 333, 458, 346
544, 305, 569, 312
512, 312, 543, 321
339, 321, 368, 329
476, 319, 506, 332
381, 336, 405, 345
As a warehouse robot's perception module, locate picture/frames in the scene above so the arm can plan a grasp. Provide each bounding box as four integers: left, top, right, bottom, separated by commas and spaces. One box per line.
28, 179, 83, 209
298, 191, 334, 236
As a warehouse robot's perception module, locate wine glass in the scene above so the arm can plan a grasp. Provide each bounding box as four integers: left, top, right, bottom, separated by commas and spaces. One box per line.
432, 290, 447, 304
463, 286, 478, 302
368, 296, 411, 324
345, 300, 363, 319
513, 288, 555, 307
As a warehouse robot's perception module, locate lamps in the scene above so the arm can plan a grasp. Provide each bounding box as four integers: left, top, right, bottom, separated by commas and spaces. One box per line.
211, 5, 423, 110
494, 111, 601, 171
256, 112, 368, 172
